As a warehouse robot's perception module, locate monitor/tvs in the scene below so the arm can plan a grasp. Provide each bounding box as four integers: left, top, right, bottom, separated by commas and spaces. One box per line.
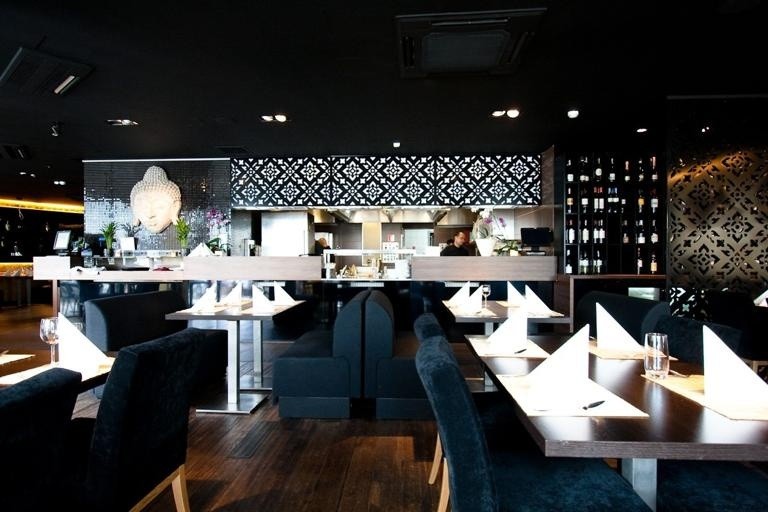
53, 230, 71, 255
521, 227, 552, 252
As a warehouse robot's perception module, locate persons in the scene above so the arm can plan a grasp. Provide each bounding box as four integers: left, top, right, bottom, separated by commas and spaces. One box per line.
129, 164, 183, 234
440, 230, 471, 256
447, 239, 454, 246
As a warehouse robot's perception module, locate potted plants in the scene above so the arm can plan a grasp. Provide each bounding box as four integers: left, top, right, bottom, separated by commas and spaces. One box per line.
206, 237, 233, 256
475, 224, 519, 256
99, 220, 142, 257
172, 217, 194, 256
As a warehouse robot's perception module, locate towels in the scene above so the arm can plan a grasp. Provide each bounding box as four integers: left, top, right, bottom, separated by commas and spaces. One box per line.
0, 311, 112, 385
176, 280, 305, 316
443, 279, 564, 317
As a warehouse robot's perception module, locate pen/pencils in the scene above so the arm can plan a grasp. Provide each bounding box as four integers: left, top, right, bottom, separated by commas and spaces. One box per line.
515, 348, 527, 354
584, 400, 604, 410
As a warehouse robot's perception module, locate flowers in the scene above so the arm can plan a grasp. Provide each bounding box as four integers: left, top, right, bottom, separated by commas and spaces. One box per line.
477, 211, 506, 240
205, 208, 232, 234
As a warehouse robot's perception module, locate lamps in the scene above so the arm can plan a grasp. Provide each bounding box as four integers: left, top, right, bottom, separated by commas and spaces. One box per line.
32, 255, 71, 316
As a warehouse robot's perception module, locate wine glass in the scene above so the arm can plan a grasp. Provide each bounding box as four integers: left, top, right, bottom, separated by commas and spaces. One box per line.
482, 285, 491, 309
40, 317, 59, 365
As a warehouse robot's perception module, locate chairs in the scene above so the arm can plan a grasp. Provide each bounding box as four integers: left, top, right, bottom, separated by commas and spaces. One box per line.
71, 333, 192, 511
657, 454, 768, 512
576, 292, 767, 374
411, 312, 605, 486
0, 370, 81, 511
413, 335, 652, 512
442, 299, 571, 392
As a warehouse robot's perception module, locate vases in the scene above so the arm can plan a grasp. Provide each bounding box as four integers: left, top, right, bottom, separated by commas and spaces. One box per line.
209, 232, 228, 256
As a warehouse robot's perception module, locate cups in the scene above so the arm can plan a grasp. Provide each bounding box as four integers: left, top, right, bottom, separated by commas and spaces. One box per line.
644, 333, 670, 380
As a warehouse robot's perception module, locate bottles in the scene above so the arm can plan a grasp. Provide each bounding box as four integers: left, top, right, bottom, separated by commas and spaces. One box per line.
566, 157, 630, 181
637, 156, 658, 275
581, 250, 589, 274
566, 250, 573, 274
596, 250, 602, 273
566, 187, 573, 213
622, 220, 629, 244
581, 186, 627, 214
568, 219, 605, 244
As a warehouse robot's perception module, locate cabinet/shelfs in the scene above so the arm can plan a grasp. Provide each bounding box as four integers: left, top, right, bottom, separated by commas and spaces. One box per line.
552, 155, 674, 333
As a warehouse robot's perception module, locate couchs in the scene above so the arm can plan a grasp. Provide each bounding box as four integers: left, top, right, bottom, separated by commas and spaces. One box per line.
83, 287, 188, 400
271, 289, 436, 419
